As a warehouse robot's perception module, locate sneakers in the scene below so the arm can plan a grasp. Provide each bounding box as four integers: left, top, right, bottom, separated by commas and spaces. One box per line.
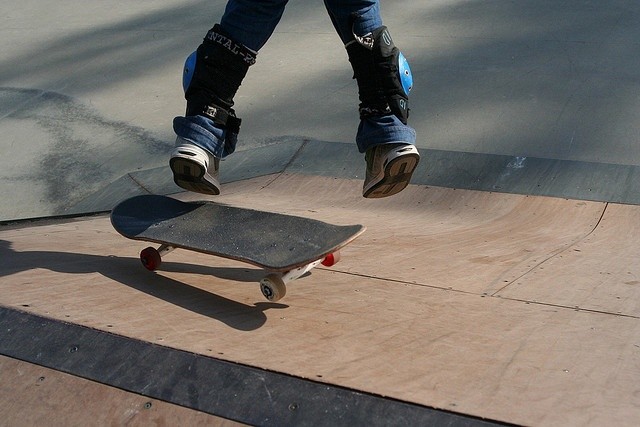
363, 144, 420, 198
169, 136, 221, 195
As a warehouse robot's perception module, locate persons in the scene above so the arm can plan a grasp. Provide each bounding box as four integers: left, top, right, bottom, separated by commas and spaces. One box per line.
169, 1, 420, 198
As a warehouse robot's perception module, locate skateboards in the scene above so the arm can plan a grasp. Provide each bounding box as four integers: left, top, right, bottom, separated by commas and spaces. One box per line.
110, 194, 367, 302
